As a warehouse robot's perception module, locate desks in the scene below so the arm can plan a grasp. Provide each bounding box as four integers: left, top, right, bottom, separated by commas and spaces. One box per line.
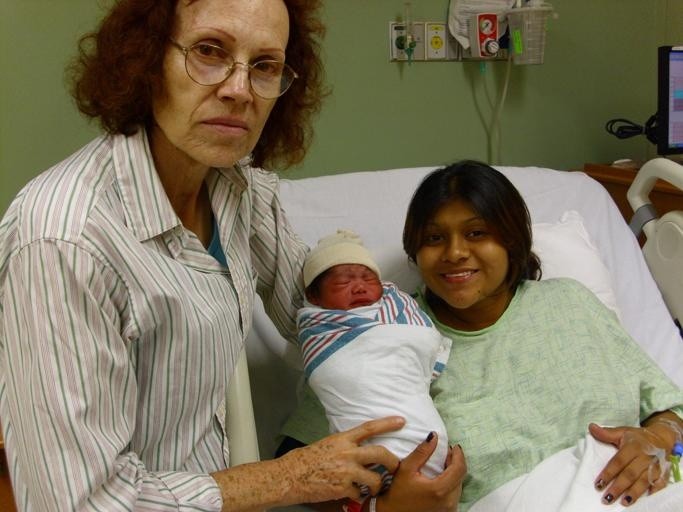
572, 159, 683, 249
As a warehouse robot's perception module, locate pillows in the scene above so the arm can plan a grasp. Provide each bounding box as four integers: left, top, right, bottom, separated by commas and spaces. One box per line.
370, 210, 625, 324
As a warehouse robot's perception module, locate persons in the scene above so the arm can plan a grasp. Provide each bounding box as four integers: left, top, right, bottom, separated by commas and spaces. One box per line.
302, 230, 449, 492
0, 0, 407, 511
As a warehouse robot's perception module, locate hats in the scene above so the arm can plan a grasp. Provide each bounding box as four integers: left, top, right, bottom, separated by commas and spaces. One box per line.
303, 229, 381, 289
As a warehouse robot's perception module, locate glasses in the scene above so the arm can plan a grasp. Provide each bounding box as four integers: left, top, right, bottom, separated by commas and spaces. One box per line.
172, 41, 298, 99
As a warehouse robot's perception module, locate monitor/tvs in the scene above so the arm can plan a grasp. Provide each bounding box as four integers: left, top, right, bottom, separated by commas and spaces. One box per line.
658, 45, 683, 156
